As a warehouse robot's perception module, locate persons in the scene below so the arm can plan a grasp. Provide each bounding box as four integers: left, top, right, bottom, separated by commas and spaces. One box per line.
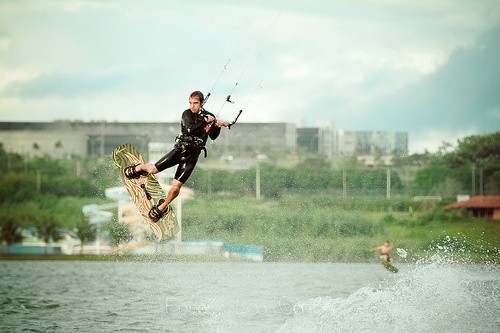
374, 241, 394, 265
124, 91, 223, 218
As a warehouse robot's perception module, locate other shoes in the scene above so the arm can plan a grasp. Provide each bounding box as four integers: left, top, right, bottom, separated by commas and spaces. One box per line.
124, 164, 144, 179
148, 198, 170, 222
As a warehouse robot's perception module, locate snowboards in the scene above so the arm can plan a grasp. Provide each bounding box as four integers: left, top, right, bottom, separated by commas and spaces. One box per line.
112, 143, 181, 242
381, 260, 399, 274
